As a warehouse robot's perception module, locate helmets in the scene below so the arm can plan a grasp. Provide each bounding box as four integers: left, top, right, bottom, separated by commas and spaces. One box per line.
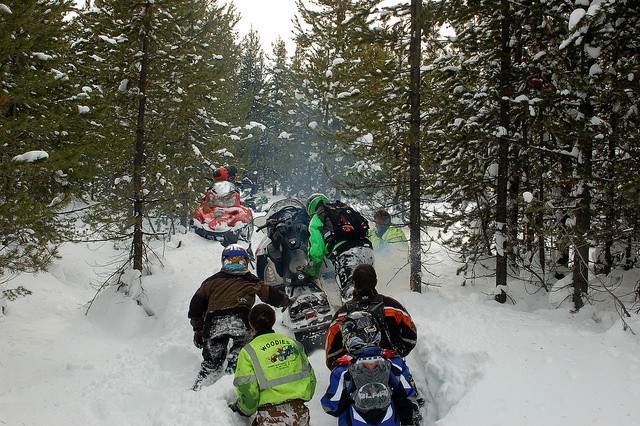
341, 310, 381, 351
306, 192, 329, 211
221, 244, 250, 265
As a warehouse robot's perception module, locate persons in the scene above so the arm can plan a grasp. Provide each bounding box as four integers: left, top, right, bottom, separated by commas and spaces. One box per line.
205, 165, 241, 206
228, 304, 316, 426
305, 193, 375, 306
366, 210, 407, 248
321, 310, 425, 426
326, 264, 416, 371
188, 244, 296, 391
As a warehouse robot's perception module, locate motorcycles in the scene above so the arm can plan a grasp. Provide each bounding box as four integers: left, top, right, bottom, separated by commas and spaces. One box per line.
190, 180, 254, 248
252, 198, 336, 354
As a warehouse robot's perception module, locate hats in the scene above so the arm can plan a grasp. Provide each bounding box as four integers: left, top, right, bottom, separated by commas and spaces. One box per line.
351, 265, 377, 281
248, 303, 275, 328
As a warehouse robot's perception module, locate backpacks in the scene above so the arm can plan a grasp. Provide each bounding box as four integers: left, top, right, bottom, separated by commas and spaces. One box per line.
349, 356, 394, 413
317, 200, 369, 251
